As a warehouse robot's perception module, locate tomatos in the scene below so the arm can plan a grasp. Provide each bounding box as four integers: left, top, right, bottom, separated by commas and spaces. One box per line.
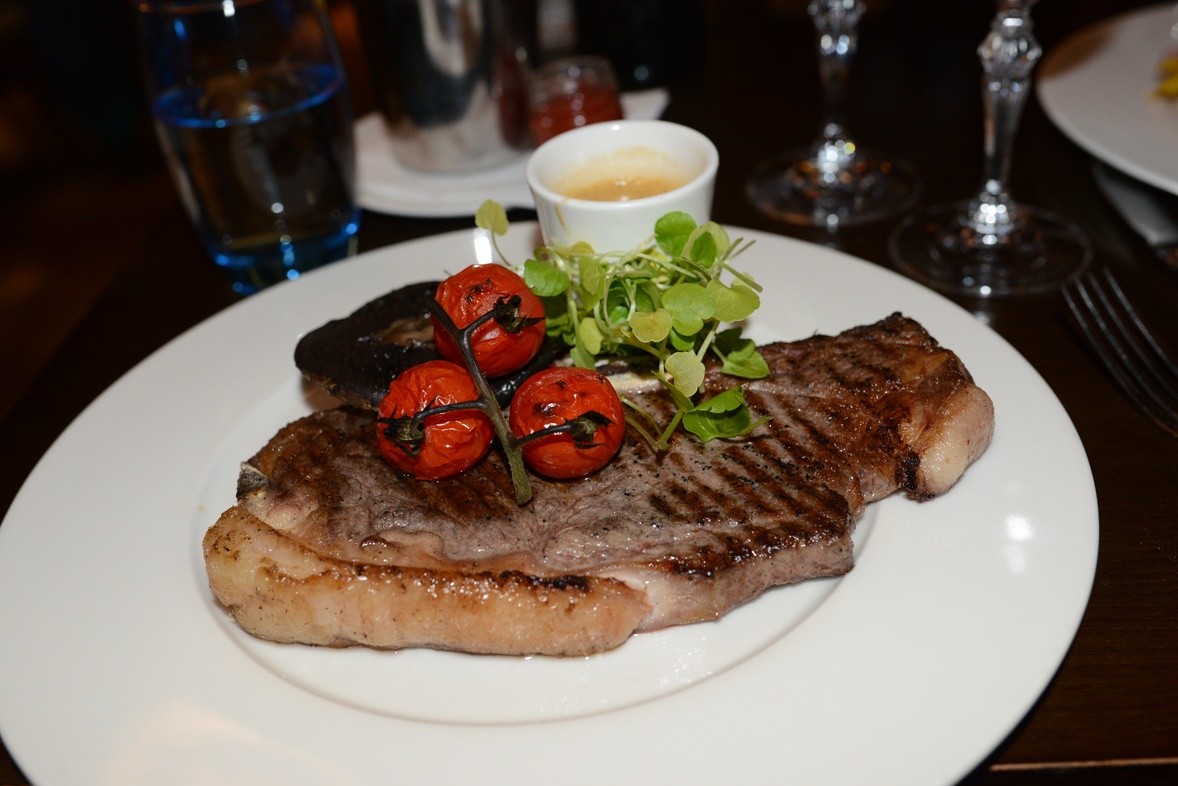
509, 363, 625, 478
376, 360, 498, 480
431, 262, 547, 375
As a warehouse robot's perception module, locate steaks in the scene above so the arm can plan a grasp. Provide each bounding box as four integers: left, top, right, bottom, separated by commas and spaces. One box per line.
294, 278, 575, 411
200, 310, 996, 658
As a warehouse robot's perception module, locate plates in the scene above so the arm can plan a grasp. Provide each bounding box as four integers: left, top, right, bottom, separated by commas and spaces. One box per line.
0, 223, 1100, 785
354, 103, 535, 219
1036, 7, 1178, 197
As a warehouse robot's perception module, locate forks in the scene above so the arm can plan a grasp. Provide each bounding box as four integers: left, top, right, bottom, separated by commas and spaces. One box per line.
1062, 268, 1177, 436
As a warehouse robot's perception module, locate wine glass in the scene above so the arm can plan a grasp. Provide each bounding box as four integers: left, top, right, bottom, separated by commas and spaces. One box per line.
744, 0, 930, 227
893, 0, 1096, 296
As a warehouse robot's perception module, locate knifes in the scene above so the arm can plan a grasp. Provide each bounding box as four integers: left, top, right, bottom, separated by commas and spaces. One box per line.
1087, 160, 1176, 274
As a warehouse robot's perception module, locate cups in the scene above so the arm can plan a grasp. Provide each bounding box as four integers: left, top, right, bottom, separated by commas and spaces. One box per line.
524, 117, 721, 267
136, 1, 359, 292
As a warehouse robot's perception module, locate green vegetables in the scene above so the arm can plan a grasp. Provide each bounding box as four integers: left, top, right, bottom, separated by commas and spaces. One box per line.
479, 199, 773, 454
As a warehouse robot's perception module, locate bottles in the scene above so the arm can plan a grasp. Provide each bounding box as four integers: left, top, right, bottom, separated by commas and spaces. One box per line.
358, 1, 492, 171
487, 1, 622, 153
